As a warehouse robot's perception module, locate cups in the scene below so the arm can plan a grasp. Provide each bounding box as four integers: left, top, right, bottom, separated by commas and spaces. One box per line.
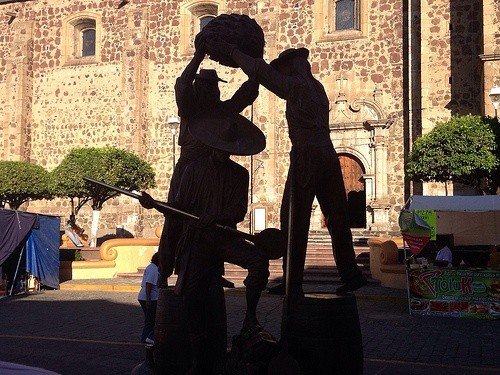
410, 264, 419, 270
417, 258, 425, 269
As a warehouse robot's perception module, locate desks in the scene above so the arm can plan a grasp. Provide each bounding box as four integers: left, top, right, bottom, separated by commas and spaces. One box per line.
404, 267, 500, 319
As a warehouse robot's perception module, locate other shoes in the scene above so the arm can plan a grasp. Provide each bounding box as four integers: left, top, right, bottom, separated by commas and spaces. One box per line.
146, 337, 154, 344
141, 340, 145, 343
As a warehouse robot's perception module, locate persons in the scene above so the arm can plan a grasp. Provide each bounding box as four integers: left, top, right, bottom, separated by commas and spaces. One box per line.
432, 238, 452, 267
204, 34, 368, 297
157, 39, 259, 287
137, 252, 159, 344
140, 110, 269, 338
